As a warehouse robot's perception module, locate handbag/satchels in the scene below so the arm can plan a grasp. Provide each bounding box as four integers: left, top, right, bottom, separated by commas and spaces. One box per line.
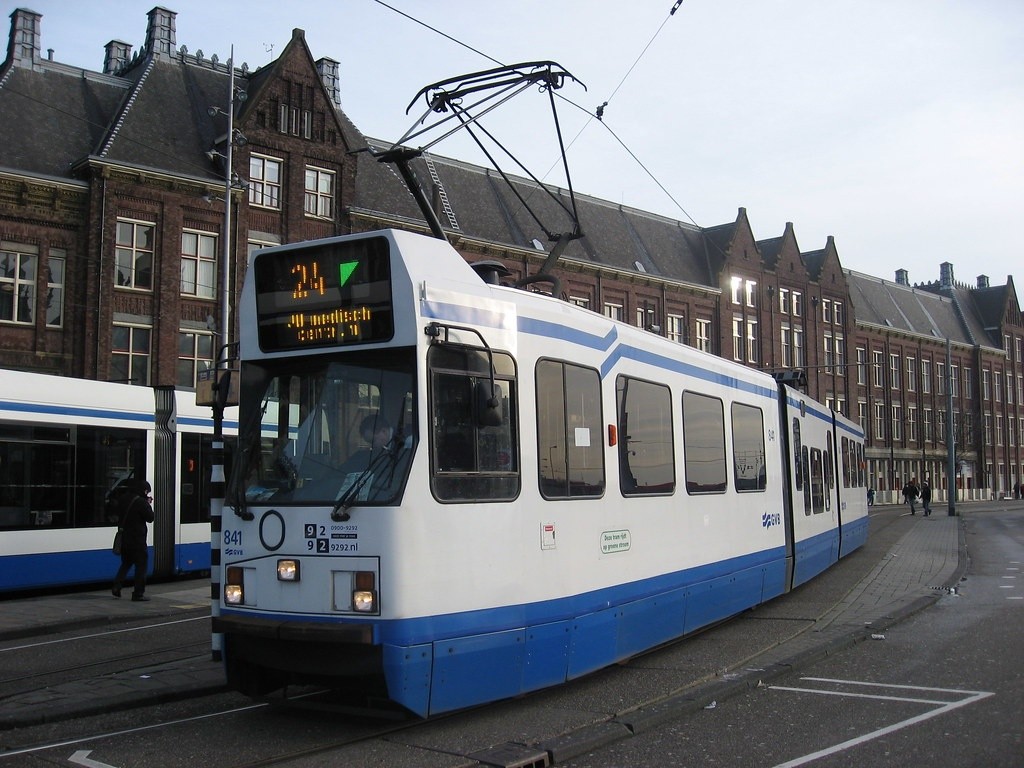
113, 532, 123, 556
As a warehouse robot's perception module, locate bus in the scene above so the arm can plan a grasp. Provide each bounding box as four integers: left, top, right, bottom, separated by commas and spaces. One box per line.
0, 367, 331, 598
216, 226, 872, 720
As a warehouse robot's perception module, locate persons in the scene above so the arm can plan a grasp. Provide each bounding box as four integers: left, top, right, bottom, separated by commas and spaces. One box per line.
359, 413, 413, 449
111, 481, 154, 602
867, 487, 875, 506
902, 481, 931, 516
1014, 482, 1024, 500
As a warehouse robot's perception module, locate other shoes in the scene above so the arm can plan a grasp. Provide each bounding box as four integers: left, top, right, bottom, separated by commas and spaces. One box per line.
929, 510, 931, 515
923, 515, 928, 516
112, 588, 121, 597
132, 592, 149, 601
